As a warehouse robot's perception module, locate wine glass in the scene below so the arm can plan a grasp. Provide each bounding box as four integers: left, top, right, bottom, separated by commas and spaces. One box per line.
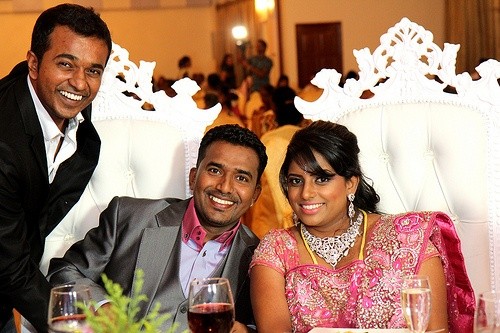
48, 284, 96, 333
187, 278, 235, 333
472, 292, 500, 333
400, 275, 433, 333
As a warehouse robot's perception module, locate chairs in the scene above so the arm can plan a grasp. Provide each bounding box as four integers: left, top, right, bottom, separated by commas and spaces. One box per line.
20, 36, 223, 333
291, 16, 499, 333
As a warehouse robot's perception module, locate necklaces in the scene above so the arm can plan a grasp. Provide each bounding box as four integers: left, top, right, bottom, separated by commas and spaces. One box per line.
296, 204, 368, 271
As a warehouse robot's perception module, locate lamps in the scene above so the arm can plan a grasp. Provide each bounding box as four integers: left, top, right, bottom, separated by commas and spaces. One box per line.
253, 0, 277, 18
231, 25, 247, 47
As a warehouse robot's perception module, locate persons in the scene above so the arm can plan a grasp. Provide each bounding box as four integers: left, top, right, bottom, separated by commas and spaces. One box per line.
1, 2, 112, 330
246, 120, 476, 332
42, 122, 262, 332
163, 39, 314, 124
